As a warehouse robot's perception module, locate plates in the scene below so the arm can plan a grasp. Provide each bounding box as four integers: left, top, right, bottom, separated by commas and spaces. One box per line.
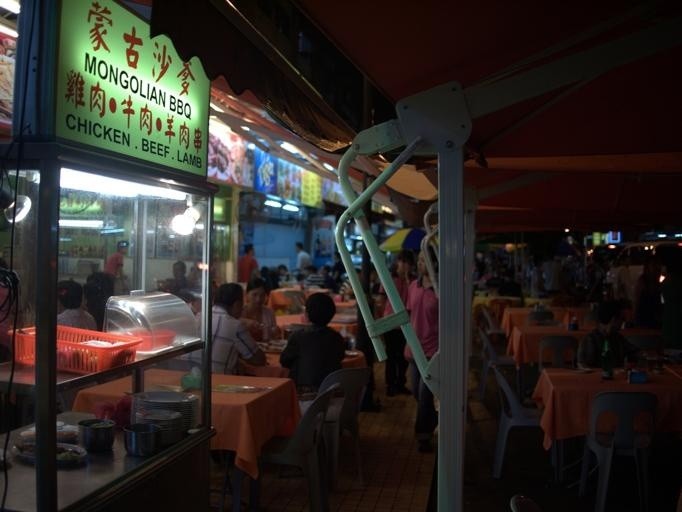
23, 424, 80, 443
12, 443, 87, 467
130, 391, 200, 427
135, 408, 182, 444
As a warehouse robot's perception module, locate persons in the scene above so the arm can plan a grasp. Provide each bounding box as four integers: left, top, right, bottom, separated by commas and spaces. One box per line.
236, 242, 262, 283
276, 239, 363, 301
81, 271, 114, 330
278, 292, 347, 395
103, 239, 129, 296
528, 243, 682, 370
377, 256, 410, 398
177, 280, 269, 377
237, 277, 276, 344
50, 278, 98, 336
404, 245, 441, 452
162, 260, 188, 292
472, 250, 495, 282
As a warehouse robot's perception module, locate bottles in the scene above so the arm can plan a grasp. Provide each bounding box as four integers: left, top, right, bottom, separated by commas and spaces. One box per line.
601, 340, 614, 380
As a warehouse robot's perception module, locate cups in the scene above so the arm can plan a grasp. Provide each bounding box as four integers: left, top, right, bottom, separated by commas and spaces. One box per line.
266, 328, 279, 347
636, 353, 648, 370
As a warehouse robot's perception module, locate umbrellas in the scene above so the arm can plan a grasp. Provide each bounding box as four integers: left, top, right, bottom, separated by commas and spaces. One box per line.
378, 226, 438, 253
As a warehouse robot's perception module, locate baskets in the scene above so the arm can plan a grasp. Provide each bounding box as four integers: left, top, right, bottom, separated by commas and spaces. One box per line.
6, 325, 144, 375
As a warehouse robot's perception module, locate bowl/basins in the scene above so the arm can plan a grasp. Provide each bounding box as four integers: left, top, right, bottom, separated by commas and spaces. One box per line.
123, 424, 161, 457
79, 420, 115, 452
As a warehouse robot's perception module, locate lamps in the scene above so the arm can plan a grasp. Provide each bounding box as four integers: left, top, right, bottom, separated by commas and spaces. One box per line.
0, 167, 32, 225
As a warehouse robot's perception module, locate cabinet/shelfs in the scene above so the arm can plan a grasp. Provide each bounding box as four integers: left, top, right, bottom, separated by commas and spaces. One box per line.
0, 141, 214, 512
